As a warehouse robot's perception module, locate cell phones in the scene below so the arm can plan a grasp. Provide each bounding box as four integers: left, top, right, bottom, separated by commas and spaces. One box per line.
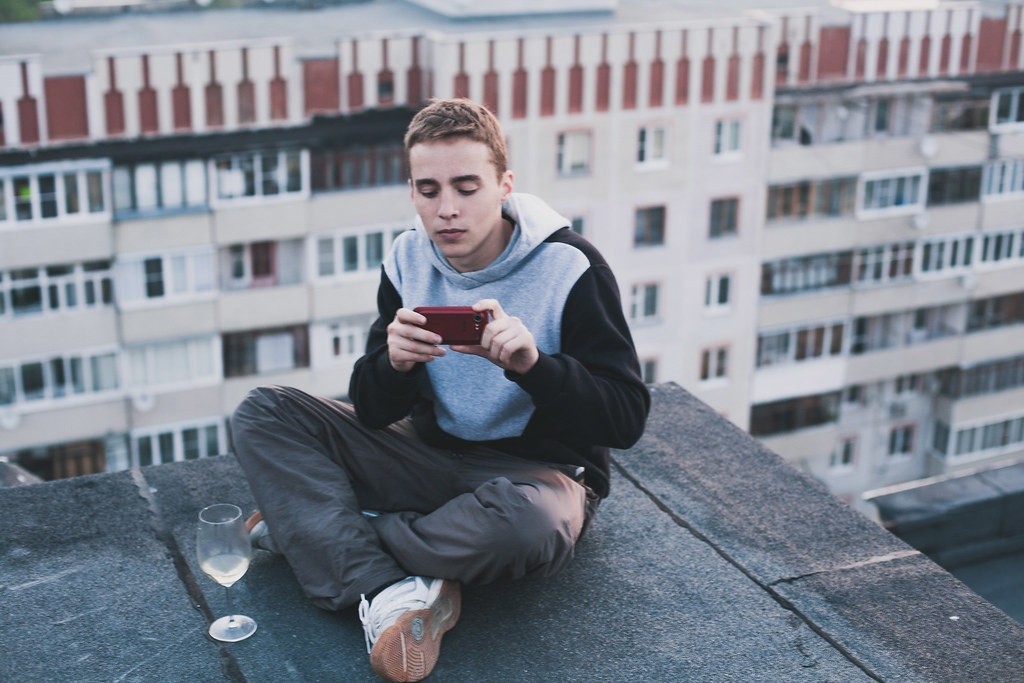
413, 307, 490, 346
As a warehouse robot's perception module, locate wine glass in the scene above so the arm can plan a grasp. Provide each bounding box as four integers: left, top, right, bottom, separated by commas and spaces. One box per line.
196, 503, 258, 642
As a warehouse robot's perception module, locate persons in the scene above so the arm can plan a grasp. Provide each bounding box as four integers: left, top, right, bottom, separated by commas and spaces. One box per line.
224, 99, 653, 682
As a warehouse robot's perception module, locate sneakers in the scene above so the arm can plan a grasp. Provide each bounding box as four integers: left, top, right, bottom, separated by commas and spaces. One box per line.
245, 511, 281, 556
357, 575, 461, 682
